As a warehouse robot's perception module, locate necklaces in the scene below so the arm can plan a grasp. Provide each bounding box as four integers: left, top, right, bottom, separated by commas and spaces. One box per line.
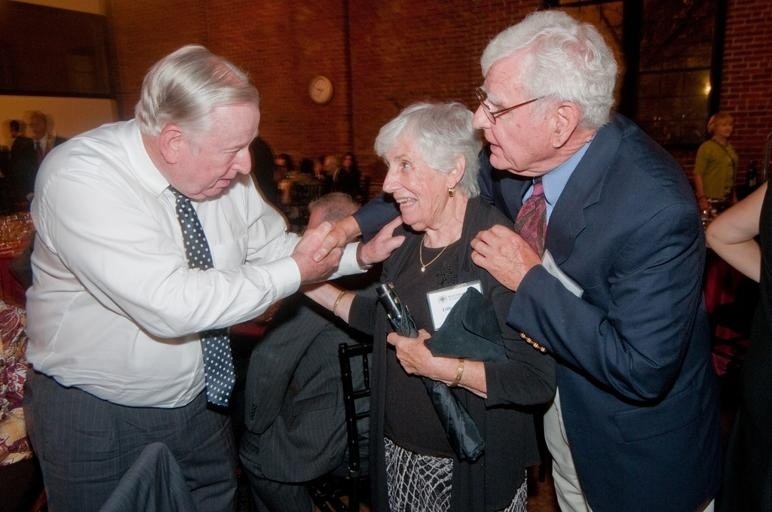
418, 233, 452, 273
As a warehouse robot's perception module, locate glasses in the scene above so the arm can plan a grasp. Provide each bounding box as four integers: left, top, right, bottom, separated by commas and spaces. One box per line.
475, 86, 558, 124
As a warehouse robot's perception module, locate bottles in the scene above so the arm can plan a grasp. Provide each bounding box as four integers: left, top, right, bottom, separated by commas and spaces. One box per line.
747, 159, 757, 191
652, 115, 664, 142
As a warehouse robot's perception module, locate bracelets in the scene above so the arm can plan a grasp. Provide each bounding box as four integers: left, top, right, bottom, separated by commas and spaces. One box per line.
333, 290, 347, 314
356, 244, 373, 270
447, 357, 465, 389
698, 194, 706, 200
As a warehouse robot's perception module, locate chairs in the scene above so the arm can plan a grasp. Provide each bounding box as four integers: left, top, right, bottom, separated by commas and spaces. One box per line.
337, 341, 374, 512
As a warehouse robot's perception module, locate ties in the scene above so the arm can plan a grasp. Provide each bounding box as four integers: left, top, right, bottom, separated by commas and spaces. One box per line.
514, 183, 548, 263
36, 139, 45, 168
166, 185, 237, 407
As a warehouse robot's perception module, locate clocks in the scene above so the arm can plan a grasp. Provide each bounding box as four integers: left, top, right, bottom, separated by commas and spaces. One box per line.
309, 76, 333, 105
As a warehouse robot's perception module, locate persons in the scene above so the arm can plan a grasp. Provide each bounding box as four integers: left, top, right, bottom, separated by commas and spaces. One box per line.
704, 175, 772, 510
27, 110, 69, 194
271, 151, 358, 231
236, 193, 361, 511
311, 11, 718, 511
9, 118, 32, 199
693, 110, 738, 213
25, 45, 403, 511
302, 103, 557, 511
